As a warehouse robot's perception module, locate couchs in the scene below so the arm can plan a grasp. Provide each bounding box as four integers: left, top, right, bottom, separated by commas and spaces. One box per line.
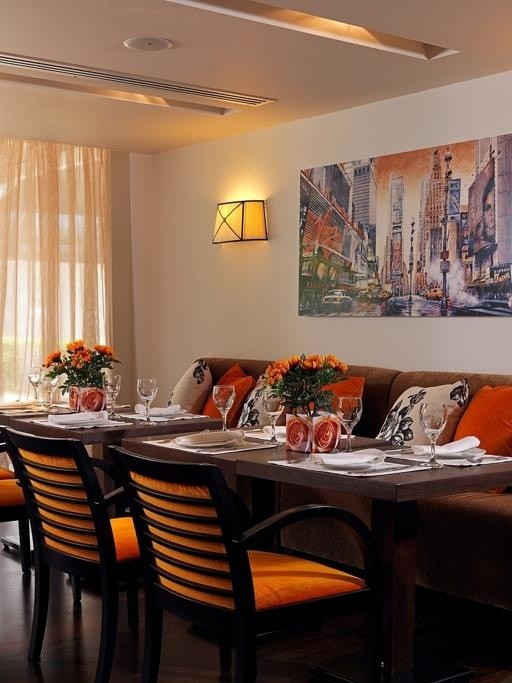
188, 358, 512, 615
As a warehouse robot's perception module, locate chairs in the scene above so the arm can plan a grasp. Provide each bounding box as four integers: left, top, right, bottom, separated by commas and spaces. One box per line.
1, 421, 37, 578
2, 423, 261, 682
105, 437, 386, 682
1, 442, 20, 480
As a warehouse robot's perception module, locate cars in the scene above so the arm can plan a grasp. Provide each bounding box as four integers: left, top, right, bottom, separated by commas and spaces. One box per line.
419, 284, 443, 299
359, 289, 391, 299
322, 288, 351, 311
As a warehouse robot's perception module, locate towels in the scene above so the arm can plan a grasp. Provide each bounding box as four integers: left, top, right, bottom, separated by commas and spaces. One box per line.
262, 422, 287, 435
147, 402, 184, 417
303, 443, 386, 467
46, 406, 110, 425
410, 434, 482, 456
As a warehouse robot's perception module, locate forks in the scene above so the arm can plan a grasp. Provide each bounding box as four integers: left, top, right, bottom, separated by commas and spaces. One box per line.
464, 453, 506, 464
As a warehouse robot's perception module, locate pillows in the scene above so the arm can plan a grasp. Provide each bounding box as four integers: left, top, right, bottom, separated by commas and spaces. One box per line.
199, 360, 256, 430
375, 375, 470, 449
166, 357, 215, 414
307, 374, 369, 436
453, 380, 512, 497
236, 368, 291, 428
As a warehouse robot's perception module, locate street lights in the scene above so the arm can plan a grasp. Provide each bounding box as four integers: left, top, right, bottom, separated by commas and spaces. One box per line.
441, 151, 453, 309
408, 221, 416, 301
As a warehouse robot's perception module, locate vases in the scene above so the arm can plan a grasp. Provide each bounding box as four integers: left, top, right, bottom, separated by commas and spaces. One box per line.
66, 383, 111, 413
281, 409, 341, 454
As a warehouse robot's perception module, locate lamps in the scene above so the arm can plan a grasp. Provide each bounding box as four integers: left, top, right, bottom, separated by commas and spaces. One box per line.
207, 197, 271, 247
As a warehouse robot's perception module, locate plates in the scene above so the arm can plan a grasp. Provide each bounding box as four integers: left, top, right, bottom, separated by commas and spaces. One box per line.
172, 440, 240, 447
431, 446, 484, 457
51, 418, 102, 426
149, 409, 188, 416
317, 462, 376, 471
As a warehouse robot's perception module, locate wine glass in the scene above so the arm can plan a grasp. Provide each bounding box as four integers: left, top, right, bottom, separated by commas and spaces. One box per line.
103, 374, 123, 419
27, 366, 60, 411
419, 402, 447, 470
263, 386, 286, 446
211, 384, 235, 435
338, 396, 362, 455
137, 378, 157, 425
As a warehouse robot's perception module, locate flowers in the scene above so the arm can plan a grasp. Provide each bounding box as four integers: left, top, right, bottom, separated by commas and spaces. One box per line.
263, 352, 352, 416
39, 337, 124, 395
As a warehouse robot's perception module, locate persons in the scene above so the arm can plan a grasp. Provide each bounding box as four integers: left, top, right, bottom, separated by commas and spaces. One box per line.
473, 176, 494, 254
